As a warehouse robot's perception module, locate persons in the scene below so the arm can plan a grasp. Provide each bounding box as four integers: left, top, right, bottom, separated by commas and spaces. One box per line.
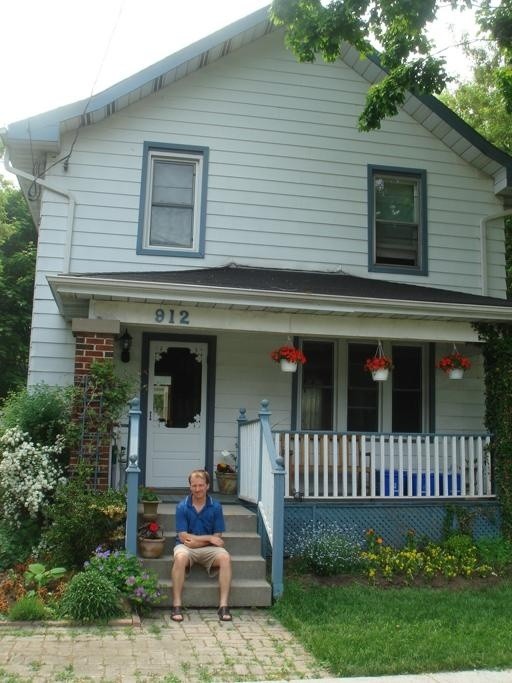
170, 471, 234, 621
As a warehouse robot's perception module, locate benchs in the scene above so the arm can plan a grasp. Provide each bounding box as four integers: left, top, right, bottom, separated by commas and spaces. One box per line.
278, 432, 372, 495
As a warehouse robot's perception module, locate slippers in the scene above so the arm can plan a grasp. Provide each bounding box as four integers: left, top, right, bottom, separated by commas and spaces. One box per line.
217, 605, 232, 621
170, 604, 183, 622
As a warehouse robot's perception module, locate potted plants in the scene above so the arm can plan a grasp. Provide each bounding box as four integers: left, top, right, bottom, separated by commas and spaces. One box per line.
140, 485, 162, 514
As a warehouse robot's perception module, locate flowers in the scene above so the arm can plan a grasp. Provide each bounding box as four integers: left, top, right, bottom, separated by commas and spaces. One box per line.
435, 350, 471, 371
364, 355, 396, 373
215, 447, 238, 471
268, 345, 306, 364
139, 517, 165, 539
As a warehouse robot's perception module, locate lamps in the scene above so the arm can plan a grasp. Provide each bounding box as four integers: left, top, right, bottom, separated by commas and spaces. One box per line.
117, 325, 134, 364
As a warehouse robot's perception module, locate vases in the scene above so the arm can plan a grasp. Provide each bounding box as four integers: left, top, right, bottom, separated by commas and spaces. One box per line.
449, 367, 466, 381
372, 368, 389, 381
279, 361, 298, 372
217, 471, 238, 494
140, 538, 165, 558
